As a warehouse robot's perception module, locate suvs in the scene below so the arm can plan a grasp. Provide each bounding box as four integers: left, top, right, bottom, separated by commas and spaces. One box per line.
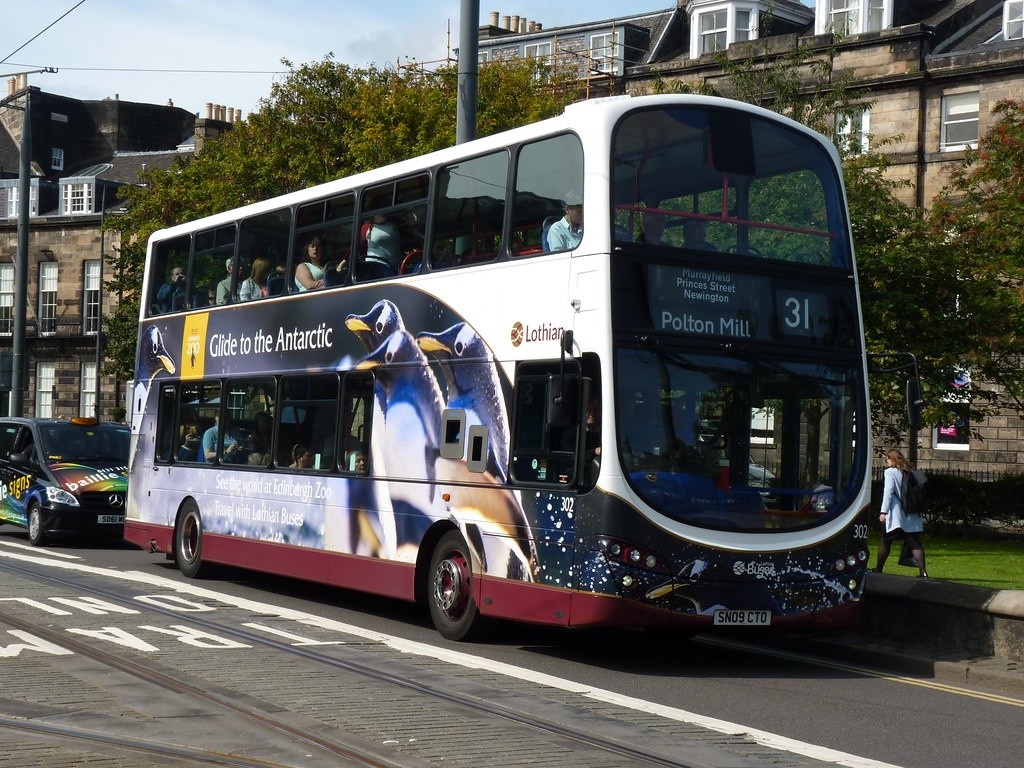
1, 416, 130, 545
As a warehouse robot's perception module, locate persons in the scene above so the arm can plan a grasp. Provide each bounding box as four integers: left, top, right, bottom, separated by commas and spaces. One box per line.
630, 206, 674, 248
679, 219, 712, 253
149, 184, 593, 314
17, 429, 71, 461
865, 448, 930, 578
178, 404, 366, 476
584, 399, 602, 463
76, 431, 111, 459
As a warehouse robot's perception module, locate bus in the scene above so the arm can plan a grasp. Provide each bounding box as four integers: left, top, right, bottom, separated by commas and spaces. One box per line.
126, 94, 872, 640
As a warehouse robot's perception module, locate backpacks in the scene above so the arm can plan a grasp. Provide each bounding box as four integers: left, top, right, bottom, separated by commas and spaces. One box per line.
900, 470, 926, 517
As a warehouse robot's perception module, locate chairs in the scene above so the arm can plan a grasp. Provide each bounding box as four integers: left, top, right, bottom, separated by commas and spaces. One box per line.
319, 259, 347, 289
542, 214, 565, 253
172, 288, 201, 312
265, 269, 287, 297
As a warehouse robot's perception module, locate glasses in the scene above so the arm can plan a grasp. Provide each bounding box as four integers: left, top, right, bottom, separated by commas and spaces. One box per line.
885, 457, 889, 463
